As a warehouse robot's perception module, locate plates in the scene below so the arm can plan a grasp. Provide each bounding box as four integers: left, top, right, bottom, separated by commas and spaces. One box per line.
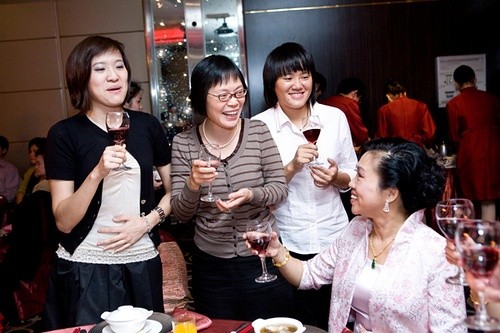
437, 161, 456, 168
87, 311, 176, 333
102, 320, 163, 333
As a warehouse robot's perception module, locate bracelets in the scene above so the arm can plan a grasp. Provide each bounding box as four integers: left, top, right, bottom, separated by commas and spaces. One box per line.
272, 246, 290, 267
152, 207, 166, 225
142, 215, 151, 232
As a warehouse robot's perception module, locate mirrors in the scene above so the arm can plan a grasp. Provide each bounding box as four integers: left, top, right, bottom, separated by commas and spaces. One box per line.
142, 0, 252, 149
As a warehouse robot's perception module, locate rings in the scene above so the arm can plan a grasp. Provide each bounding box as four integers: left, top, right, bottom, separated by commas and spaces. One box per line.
114, 153, 116, 158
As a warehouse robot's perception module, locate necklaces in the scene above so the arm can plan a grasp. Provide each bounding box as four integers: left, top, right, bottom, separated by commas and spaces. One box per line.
370, 232, 394, 269
202, 118, 238, 149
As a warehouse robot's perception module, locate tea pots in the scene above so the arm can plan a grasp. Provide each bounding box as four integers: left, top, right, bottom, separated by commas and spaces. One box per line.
435, 141, 452, 157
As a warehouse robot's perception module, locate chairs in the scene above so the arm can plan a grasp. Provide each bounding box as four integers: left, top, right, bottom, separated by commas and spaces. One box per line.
157, 228, 194, 310
0, 190, 58, 323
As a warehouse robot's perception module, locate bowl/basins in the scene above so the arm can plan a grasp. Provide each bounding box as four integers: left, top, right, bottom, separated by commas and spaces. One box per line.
251, 317, 306, 333
101, 305, 153, 333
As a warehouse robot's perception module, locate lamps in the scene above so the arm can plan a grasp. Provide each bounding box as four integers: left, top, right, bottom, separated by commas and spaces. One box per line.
214, 15, 233, 38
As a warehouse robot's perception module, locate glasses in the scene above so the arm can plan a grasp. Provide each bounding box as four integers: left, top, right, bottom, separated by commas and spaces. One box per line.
208, 88, 248, 102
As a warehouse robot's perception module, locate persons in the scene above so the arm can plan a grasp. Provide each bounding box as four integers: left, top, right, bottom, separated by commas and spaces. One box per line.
242, 137, 467, 333
446, 64, 500, 222
444, 236, 500, 303
321, 81, 370, 155
0, 35, 358, 333
373, 83, 436, 148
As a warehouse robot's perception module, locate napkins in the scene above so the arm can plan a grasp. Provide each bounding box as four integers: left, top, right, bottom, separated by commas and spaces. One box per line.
171, 307, 212, 330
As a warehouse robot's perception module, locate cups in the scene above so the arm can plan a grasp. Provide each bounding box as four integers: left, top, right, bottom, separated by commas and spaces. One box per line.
171, 313, 197, 333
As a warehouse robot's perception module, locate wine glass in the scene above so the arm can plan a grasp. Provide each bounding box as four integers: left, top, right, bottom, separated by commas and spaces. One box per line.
105, 112, 135, 171
198, 144, 222, 202
301, 115, 324, 166
246, 220, 277, 282
454, 220, 500, 332
436, 198, 475, 286
